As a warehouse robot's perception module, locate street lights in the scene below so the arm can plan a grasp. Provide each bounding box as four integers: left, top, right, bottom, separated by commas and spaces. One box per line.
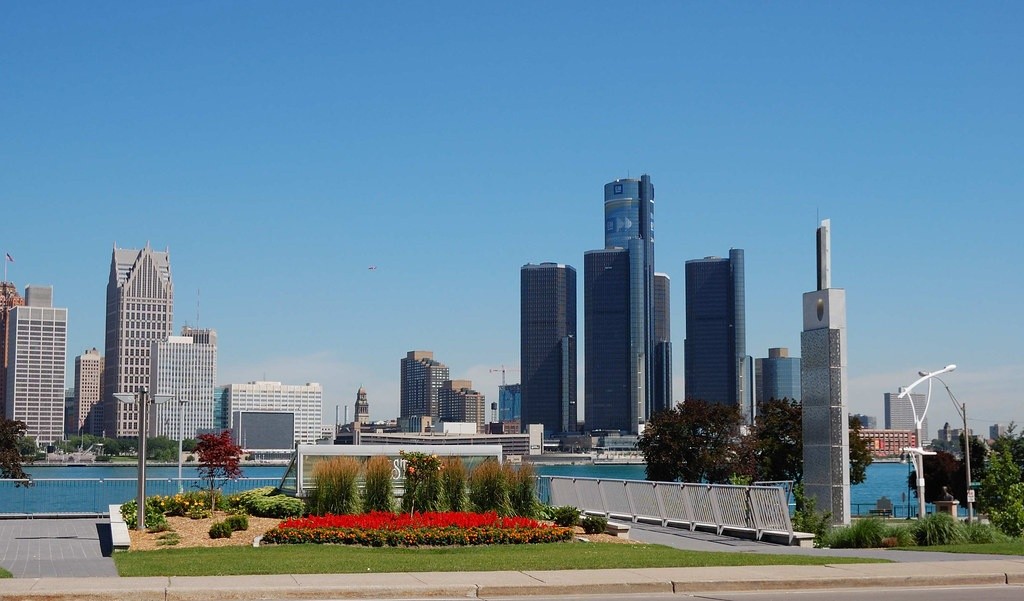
177, 399, 190, 496
112, 385, 174, 531
917, 370, 974, 524
897, 364, 959, 518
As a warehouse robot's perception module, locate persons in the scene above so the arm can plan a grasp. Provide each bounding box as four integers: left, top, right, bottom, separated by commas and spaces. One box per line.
937, 486, 953, 501
